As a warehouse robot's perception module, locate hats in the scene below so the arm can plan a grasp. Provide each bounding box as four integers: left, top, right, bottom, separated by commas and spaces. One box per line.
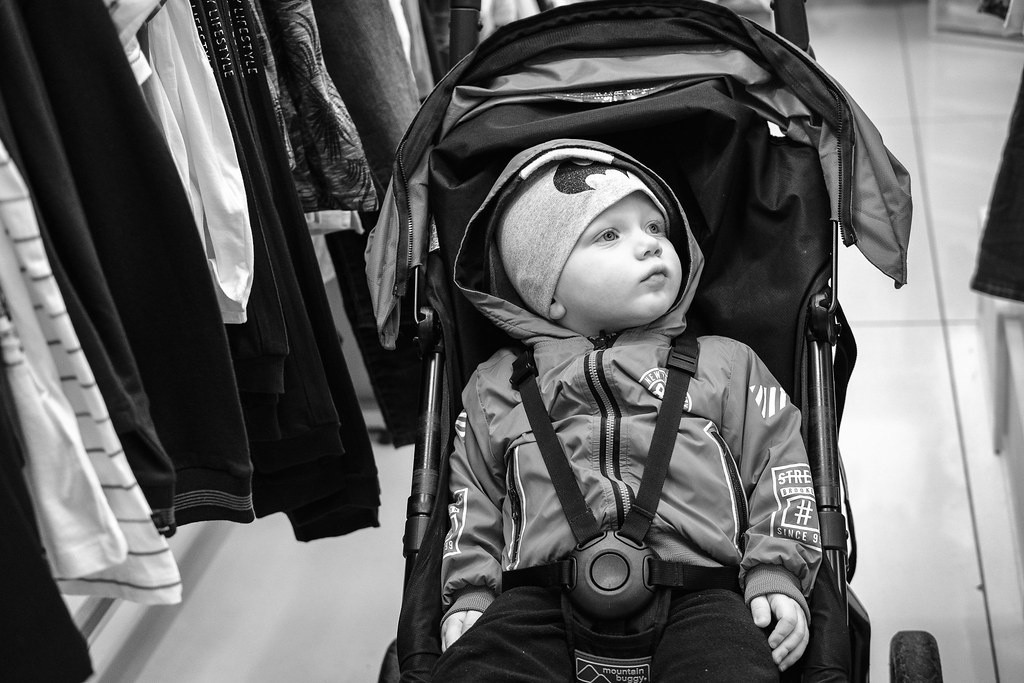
498, 157, 670, 321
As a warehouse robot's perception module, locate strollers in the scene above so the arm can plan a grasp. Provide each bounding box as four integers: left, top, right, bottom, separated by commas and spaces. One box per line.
377, 1, 945, 683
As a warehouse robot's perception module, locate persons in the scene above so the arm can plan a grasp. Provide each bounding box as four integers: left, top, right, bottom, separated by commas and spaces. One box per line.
437, 138, 824, 683
